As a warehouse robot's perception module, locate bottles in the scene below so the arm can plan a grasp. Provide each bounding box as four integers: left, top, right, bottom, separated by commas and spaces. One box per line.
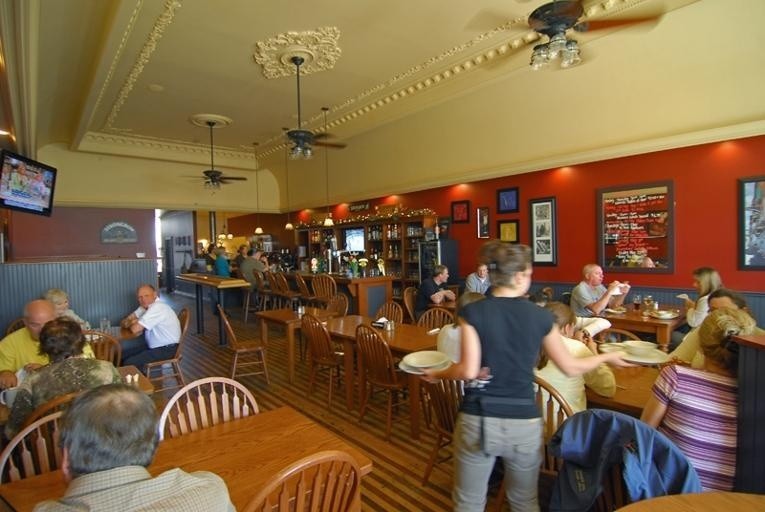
395, 244, 398, 259
101, 316, 111, 341
388, 245, 393, 259
369, 255, 374, 277
367, 226, 383, 240
374, 254, 379, 277
407, 224, 422, 236
387, 224, 401, 240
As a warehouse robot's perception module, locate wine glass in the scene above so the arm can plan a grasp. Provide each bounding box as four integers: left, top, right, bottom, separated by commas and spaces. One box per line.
644, 295, 652, 313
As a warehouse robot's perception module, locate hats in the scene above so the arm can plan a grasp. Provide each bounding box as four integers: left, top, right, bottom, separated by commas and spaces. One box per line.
248, 246, 264, 254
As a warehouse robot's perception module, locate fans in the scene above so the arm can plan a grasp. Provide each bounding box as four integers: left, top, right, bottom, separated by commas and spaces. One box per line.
201, 121, 247, 185
286, 56, 348, 151
464, 1, 665, 72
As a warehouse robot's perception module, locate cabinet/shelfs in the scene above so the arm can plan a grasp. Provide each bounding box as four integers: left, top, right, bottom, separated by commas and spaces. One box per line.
366, 214, 436, 299
293, 227, 342, 272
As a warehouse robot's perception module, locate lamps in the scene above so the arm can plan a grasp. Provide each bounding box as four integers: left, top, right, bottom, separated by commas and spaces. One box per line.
282, 127, 294, 233
288, 144, 312, 161
252, 142, 264, 235
529, 27, 583, 70
321, 106, 335, 227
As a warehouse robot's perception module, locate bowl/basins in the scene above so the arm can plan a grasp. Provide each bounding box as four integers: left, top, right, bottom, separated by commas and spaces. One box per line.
403, 350, 449, 368
623, 340, 657, 355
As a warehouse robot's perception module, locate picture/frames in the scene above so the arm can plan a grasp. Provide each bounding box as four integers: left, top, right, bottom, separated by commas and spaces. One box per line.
451, 200, 471, 224
529, 195, 557, 266
595, 180, 675, 273
477, 206, 490, 239
737, 176, 765, 271
496, 219, 519, 244
497, 186, 518, 213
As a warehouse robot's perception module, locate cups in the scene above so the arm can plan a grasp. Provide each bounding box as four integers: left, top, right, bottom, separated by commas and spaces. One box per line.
632, 295, 641, 312
0, 387, 16, 409
111, 326, 122, 338
100, 317, 111, 334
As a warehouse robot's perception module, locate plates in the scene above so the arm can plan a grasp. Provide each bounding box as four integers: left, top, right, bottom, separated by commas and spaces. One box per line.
651, 310, 679, 320
597, 340, 671, 365
397, 358, 452, 375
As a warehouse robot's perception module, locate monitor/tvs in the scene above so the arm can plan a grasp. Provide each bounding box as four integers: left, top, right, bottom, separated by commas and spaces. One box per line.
0, 147, 58, 218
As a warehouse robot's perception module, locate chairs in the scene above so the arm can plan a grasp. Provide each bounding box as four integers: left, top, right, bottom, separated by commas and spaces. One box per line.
7, 317, 27, 333
242, 450, 363, 512
296, 272, 316, 308
145, 307, 190, 390
19, 389, 83, 433
528, 375, 574, 483
598, 327, 641, 345
326, 292, 349, 314
555, 408, 701, 512
544, 287, 554, 302
375, 300, 403, 323
417, 308, 455, 329
159, 376, 260, 441
355, 323, 431, 442
0, 410, 67, 486
83, 330, 123, 368
420, 359, 462, 488
238, 272, 256, 323
277, 273, 301, 308
311, 274, 338, 308
264, 271, 281, 309
301, 312, 344, 410
253, 268, 272, 311
403, 286, 418, 321
217, 304, 271, 390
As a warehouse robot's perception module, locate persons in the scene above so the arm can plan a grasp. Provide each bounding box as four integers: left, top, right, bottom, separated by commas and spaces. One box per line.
640, 308, 761, 493
216, 247, 231, 318
4, 315, 122, 472
528, 294, 546, 308
236, 245, 249, 304
120, 284, 182, 377
532, 301, 616, 472
43, 288, 90, 331
0, 299, 95, 390
463, 262, 492, 296
206, 244, 219, 315
31, 383, 238, 512
569, 262, 629, 344
436, 292, 486, 412
665, 290, 763, 369
240, 248, 278, 310
420, 244, 640, 512
670, 267, 725, 347
1, 160, 48, 198
415, 264, 456, 321
